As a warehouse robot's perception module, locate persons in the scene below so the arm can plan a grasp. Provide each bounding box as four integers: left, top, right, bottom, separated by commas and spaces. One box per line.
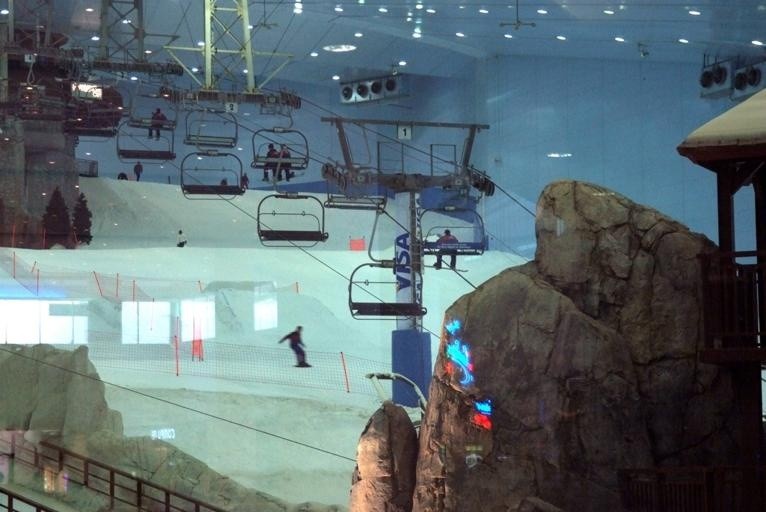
277, 143, 291, 181
434, 230, 458, 270
133, 161, 143, 181
262, 144, 280, 181
147, 108, 167, 141
241, 173, 249, 190
278, 325, 309, 367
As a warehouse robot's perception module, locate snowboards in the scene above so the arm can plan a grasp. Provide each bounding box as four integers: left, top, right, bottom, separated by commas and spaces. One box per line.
424, 266, 468, 272
